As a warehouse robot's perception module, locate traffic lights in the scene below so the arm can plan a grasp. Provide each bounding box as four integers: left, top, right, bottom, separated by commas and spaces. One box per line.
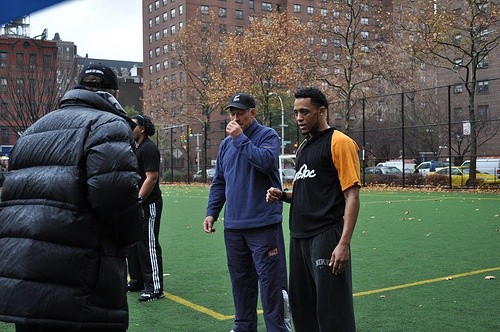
189, 128, 193, 138
184, 136, 187, 143
180, 136, 183, 143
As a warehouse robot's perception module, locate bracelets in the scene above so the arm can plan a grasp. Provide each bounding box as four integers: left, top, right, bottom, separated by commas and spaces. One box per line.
138, 197, 143, 201
279, 192, 287, 201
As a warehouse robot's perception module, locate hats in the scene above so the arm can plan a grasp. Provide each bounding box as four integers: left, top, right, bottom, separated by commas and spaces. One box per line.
78, 64, 120, 90
225, 93, 256, 111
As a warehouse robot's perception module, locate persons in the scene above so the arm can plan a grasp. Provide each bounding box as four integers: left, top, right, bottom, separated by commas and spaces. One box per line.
203, 92, 293, 332
2, 160, 7, 168
0, 63, 145, 332
266, 87, 363, 332
125, 113, 164, 304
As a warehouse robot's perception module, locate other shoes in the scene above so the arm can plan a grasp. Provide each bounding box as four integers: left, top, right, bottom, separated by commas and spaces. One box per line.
127, 282, 146, 292
138, 291, 165, 302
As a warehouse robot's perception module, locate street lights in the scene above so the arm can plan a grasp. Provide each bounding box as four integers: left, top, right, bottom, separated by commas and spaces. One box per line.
267, 91, 284, 155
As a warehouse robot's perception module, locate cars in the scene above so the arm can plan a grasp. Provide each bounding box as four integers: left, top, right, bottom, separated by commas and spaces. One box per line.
278, 154, 297, 183
192, 169, 215, 183
365, 158, 500, 187
